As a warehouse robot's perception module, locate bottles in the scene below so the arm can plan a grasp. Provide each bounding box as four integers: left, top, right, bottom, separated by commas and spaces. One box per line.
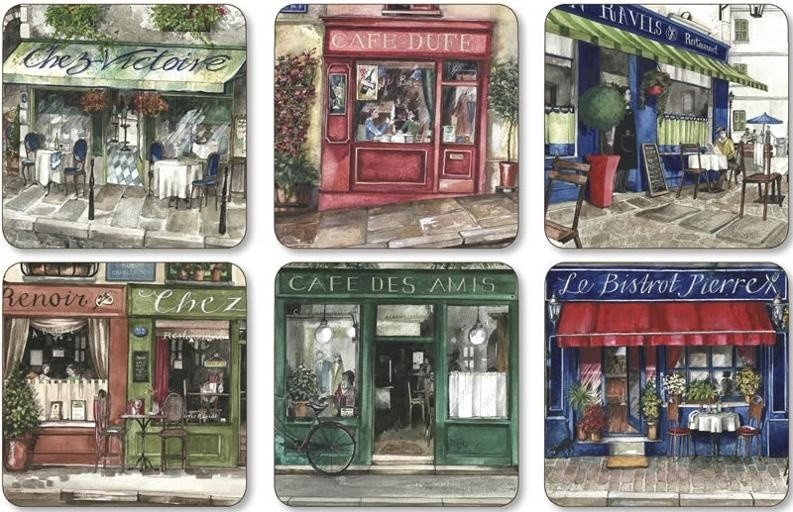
54, 132, 59, 150
360, 68, 375, 94
717, 397, 722, 414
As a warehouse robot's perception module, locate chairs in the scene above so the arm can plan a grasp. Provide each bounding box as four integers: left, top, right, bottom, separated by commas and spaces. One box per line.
186, 151, 221, 213
148, 141, 165, 199
159, 392, 188, 472
544, 155, 592, 248
406, 380, 426, 427
64, 139, 88, 201
734, 396, 765, 463
92, 395, 128, 473
21, 133, 41, 185
676, 143, 782, 221
666, 397, 693, 462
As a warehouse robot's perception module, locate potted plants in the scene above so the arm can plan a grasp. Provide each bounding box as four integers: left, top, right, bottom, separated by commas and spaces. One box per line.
485, 54, 519, 186
562, 383, 594, 441
686, 379, 719, 405
637, 70, 672, 127
735, 366, 762, 403
171, 262, 221, 282
285, 361, 319, 417
2, 358, 45, 472
580, 85, 626, 207
636, 379, 663, 441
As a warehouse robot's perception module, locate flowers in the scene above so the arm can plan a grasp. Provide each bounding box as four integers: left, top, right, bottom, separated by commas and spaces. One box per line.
661, 369, 687, 399
274, 46, 321, 198
132, 92, 169, 118
576, 403, 604, 433
80, 88, 107, 114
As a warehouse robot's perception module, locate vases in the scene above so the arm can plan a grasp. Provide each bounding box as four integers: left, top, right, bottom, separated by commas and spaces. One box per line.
588, 432, 601, 442
274, 183, 312, 217
666, 393, 683, 405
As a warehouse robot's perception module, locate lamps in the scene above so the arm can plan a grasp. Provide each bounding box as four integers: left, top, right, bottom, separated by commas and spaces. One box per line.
764, 291, 788, 337
314, 304, 333, 345
468, 305, 488, 346
544, 292, 562, 324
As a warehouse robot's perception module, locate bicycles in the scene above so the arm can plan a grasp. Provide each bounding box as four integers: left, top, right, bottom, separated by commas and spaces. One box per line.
274, 392, 357, 477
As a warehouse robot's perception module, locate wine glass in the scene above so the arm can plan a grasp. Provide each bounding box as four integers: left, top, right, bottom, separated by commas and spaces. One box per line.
133, 400, 142, 416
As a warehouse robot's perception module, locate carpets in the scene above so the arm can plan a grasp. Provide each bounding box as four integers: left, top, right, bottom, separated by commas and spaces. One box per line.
606, 455, 649, 470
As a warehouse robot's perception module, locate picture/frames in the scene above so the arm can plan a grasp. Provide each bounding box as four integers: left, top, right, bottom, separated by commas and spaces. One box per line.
48, 401, 62, 421
70, 399, 86, 421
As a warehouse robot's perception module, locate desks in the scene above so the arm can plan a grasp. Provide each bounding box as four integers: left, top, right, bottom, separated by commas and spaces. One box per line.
35, 149, 65, 197
753, 157, 788, 204
154, 159, 204, 210
106, 140, 140, 186
688, 411, 742, 473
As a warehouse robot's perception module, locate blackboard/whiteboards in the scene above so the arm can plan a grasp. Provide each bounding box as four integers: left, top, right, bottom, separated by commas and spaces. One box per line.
642, 142, 669, 197
133, 351, 149, 382
